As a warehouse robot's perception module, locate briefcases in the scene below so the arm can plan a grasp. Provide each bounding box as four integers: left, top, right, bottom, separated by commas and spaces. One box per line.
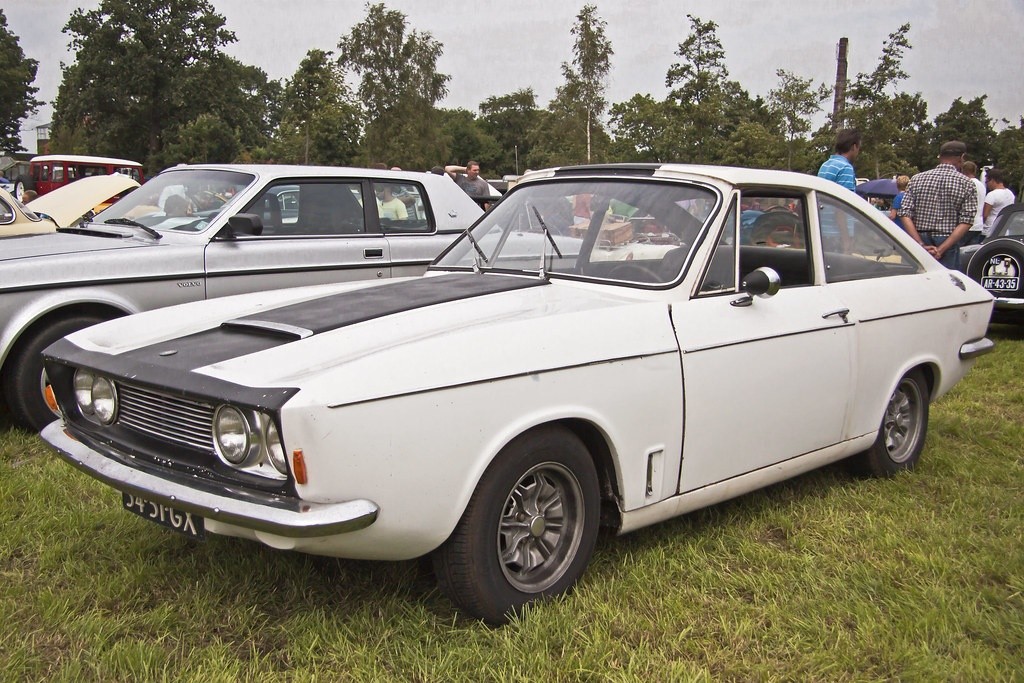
568, 220, 633, 245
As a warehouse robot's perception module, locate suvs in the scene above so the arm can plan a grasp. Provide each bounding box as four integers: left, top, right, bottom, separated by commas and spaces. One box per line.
13, 154, 147, 208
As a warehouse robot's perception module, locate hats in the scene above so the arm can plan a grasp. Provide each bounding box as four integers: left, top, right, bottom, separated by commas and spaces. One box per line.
940, 141, 974, 158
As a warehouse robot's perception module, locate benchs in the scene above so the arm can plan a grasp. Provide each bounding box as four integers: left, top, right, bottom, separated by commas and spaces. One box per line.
656, 245, 890, 286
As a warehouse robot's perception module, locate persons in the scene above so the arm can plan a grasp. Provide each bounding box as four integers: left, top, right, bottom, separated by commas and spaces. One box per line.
0, 129, 1016, 255
900, 142, 976, 276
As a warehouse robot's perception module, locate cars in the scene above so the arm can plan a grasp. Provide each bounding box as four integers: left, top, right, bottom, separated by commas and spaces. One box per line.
39, 162, 998, 627
0, 170, 166, 243
954, 203, 1024, 326
192, 185, 300, 225
0, 163, 597, 435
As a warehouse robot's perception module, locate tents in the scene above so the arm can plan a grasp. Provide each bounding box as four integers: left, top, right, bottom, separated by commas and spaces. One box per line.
857, 178, 898, 211
